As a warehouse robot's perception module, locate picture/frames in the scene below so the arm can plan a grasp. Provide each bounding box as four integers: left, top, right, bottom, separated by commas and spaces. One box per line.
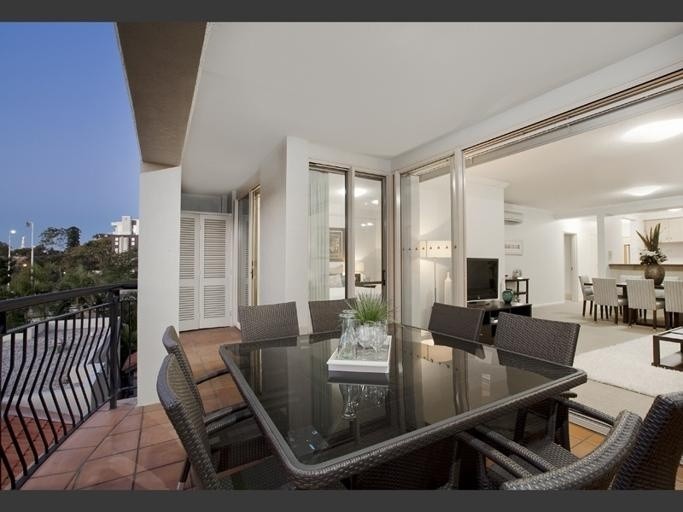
328, 227, 346, 261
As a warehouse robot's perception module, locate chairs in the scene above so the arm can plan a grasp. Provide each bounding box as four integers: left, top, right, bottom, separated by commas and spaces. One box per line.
238, 301, 300, 342
157, 325, 344, 492
427, 301, 485, 342
344, 392, 682, 492
495, 312, 583, 452
308, 297, 361, 335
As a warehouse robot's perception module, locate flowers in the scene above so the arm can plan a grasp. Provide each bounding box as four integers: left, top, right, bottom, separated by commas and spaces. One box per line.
634, 222, 667, 265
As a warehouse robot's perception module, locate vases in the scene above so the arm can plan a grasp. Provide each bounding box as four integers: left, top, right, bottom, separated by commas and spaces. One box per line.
644, 259, 665, 285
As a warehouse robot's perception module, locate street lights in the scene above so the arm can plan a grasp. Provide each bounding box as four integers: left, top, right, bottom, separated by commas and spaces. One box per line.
7, 229, 17, 290
27, 222, 34, 285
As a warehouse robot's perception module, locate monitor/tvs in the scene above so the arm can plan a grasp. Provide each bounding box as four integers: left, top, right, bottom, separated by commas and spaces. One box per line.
467, 258, 499, 305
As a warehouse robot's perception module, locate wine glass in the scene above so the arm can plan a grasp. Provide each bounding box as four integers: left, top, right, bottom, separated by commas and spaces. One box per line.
339, 309, 388, 361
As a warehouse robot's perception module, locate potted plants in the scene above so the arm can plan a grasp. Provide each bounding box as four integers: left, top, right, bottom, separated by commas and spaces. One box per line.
348, 291, 393, 349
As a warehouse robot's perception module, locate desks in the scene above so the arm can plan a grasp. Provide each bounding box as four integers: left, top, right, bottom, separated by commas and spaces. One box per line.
218, 322, 588, 488
504, 279, 529, 305
